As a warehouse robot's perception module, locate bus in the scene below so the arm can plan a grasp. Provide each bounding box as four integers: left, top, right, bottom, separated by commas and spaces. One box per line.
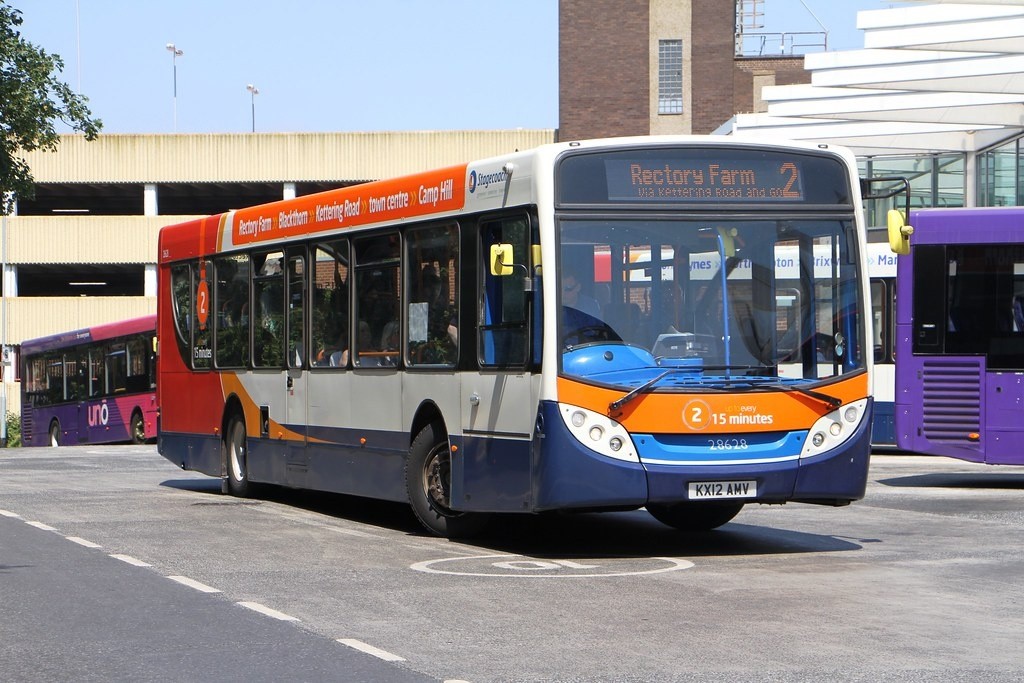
893, 205, 1024, 467
477, 228, 903, 453
153, 133, 912, 540
18, 314, 159, 448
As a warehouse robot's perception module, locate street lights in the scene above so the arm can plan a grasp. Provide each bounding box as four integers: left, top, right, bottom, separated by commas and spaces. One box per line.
246, 84, 259, 132
165, 42, 183, 133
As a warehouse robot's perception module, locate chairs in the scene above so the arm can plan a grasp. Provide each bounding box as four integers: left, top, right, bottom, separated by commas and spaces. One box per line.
1014, 294, 1024, 330
603, 302, 644, 343
